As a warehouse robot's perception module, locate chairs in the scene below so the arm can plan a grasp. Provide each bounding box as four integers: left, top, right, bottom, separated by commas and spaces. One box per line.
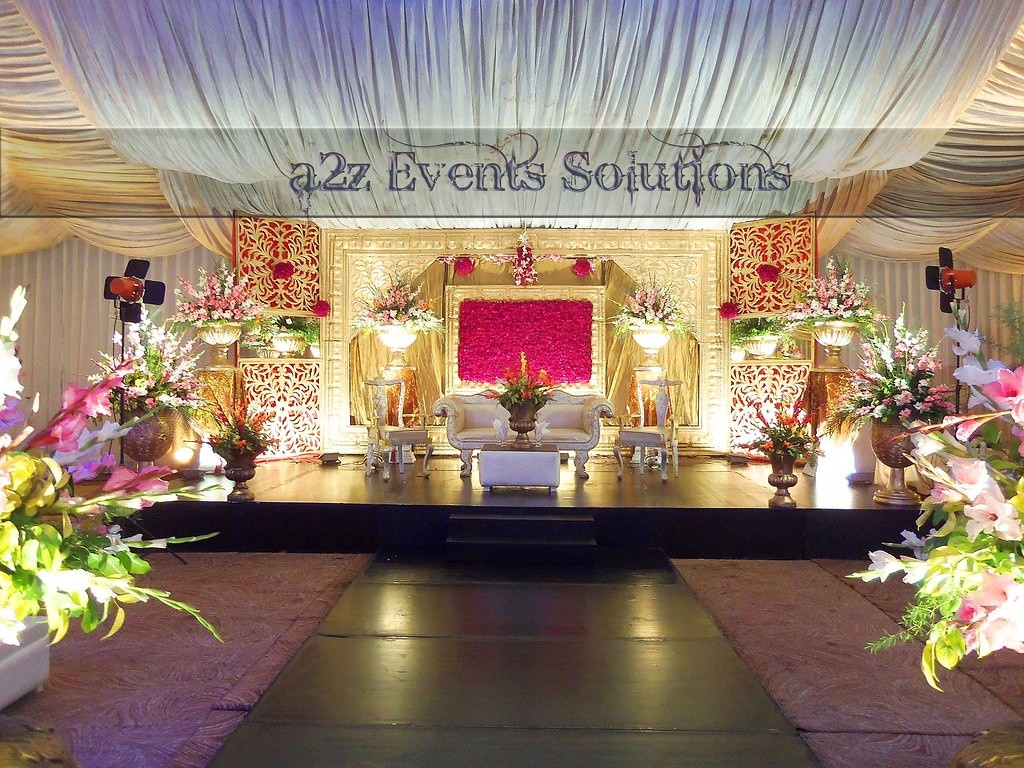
363, 378, 434, 478
614, 380, 682, 483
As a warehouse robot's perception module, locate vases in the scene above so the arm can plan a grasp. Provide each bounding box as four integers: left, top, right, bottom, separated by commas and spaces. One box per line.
379, 326, 417, 354
1, 615, 50, 711
271, 333, 304, 357
743, 336, 777, 358
506, 401, 537, 444
118, 404, 173, 470
869, 413, 925, 506
225, 450, 259, 503
809, 321, 858, 369
255, 341, 279, 358
633, 327, 669, 353
765, 452, 798, 507
201, 321, 244, 367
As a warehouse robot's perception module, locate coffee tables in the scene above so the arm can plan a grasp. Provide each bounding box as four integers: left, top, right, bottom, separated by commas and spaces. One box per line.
478, 444, 559, 495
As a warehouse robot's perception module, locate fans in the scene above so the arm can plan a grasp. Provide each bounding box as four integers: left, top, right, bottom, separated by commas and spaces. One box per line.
103, 259, 165, 466
925, 247, 978, 430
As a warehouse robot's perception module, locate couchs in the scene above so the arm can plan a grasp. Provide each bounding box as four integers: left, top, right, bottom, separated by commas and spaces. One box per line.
433, 388, 614, 478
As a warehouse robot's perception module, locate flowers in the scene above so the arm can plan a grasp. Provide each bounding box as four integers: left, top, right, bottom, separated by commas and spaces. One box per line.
601, 274, 695, 333
275, 230, 780, 319
354, 277, 448, 340
484, 351, 557, 408
731, 260, 1023, 691
0, 259, 316, 647
